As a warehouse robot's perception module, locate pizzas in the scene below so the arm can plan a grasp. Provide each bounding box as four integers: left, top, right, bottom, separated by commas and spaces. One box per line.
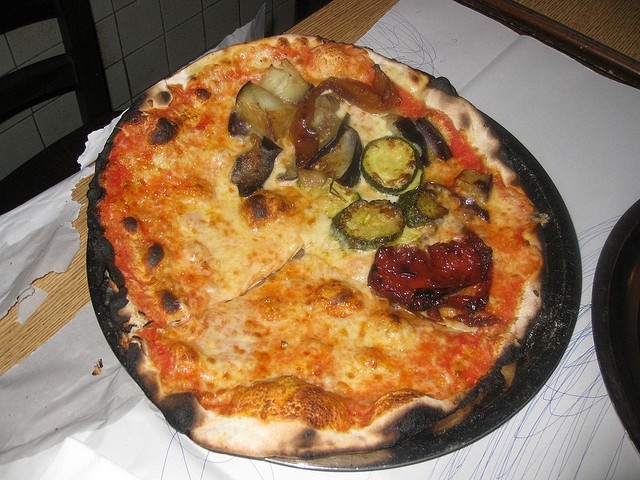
86, 34, 549, 460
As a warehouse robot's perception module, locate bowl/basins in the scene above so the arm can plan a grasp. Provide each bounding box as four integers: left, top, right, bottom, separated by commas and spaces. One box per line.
591, 198, 640, 453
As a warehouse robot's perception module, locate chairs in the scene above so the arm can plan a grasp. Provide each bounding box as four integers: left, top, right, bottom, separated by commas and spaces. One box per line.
0, 1, 124, 216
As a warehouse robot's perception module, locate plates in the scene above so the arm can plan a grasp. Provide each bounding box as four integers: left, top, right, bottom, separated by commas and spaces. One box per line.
86, 105, 582, 473
